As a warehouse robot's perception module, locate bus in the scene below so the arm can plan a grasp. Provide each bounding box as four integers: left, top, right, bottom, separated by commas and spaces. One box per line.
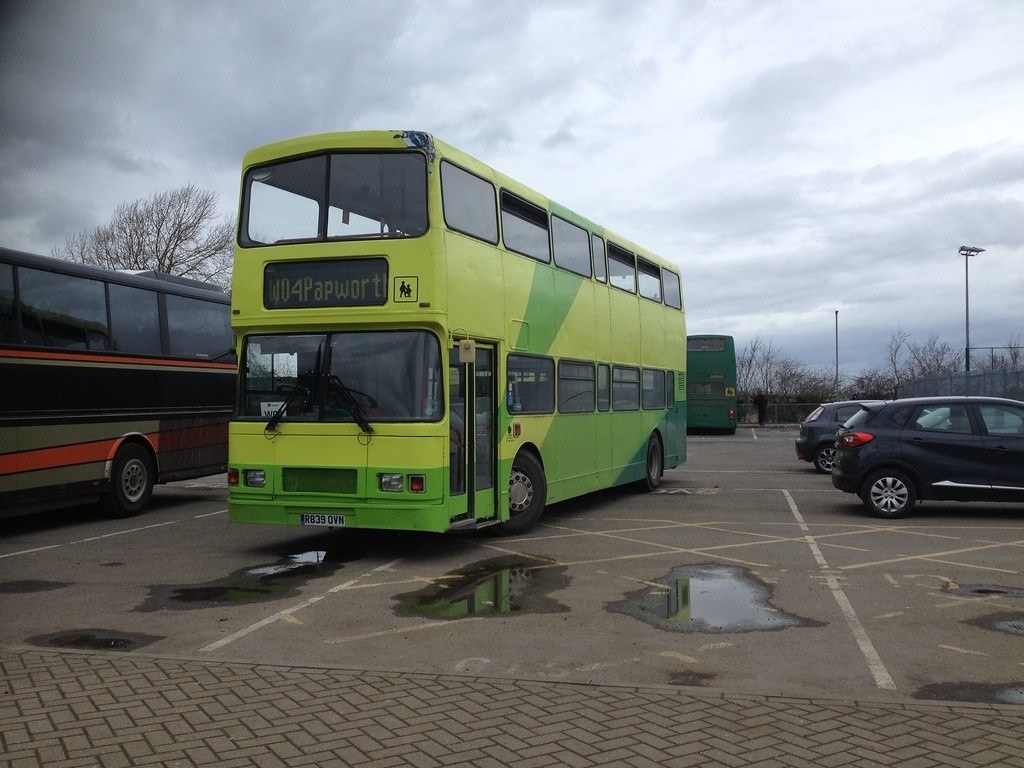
1, 243, 246, 518
650, 335, 738, 434
225, 130, 687, 533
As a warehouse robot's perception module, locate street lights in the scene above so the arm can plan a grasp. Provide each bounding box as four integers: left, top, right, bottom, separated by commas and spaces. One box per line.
958, 245, 987, 373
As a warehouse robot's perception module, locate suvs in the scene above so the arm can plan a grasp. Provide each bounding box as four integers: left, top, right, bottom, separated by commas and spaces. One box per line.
831, 395, 1024, 519
795, 400, 893, 473
